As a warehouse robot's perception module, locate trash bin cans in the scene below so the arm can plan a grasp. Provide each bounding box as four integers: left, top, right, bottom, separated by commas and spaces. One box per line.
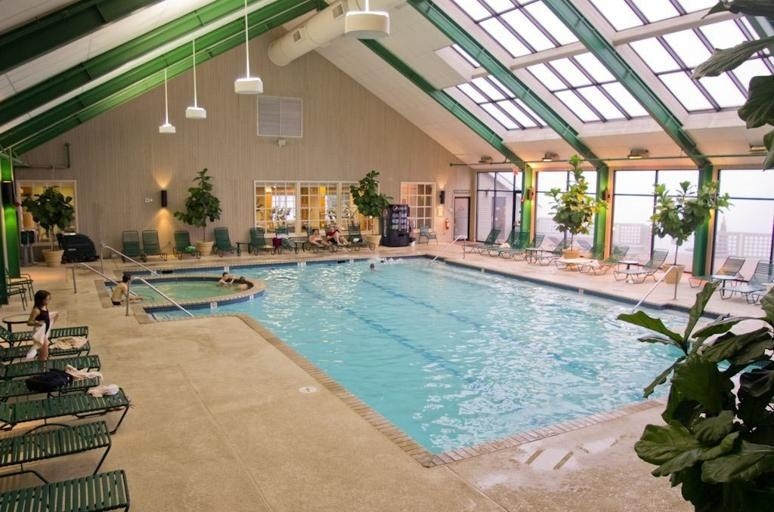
408, 237, 416, 247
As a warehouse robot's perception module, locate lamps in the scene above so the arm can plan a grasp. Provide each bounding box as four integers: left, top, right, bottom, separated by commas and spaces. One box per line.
158, 68, 177, 135
345, 1, 392, 38
185, 36, 209, 120
234, 0, 264, 96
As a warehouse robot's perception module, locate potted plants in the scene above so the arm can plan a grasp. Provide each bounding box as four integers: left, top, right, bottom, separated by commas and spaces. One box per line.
648, 178, 735, 285
349, 169, 394, 248
173, 167, 223, 256
20, 185, 76, 267
545, 153, 610, 270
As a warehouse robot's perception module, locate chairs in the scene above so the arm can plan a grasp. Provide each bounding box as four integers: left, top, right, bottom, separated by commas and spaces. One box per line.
461, 228, 631, 276
0, 283, 28, 312
719, 260, 774, 304
0, 323, 133, 512
612, 248, 669, 285
419, 223, 439, 247
687, 254, 746, 289
119, 223, 376, 264
2, 268, 35, 302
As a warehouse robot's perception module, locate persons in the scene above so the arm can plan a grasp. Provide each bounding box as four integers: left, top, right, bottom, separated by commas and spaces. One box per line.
368, 263, 378, 274
28, 290, 59, 372
216, 272, 235, 287
236, 276, 248, 289
381, 260, 384, 264
326, 226, 345, 246
308, 228, 330, 249
110, 274, 143, 305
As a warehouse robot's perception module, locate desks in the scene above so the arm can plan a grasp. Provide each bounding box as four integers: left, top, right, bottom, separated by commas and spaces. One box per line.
617, 260, 638, 278
711, 273, 737, 296
2, 314, 30, 332
